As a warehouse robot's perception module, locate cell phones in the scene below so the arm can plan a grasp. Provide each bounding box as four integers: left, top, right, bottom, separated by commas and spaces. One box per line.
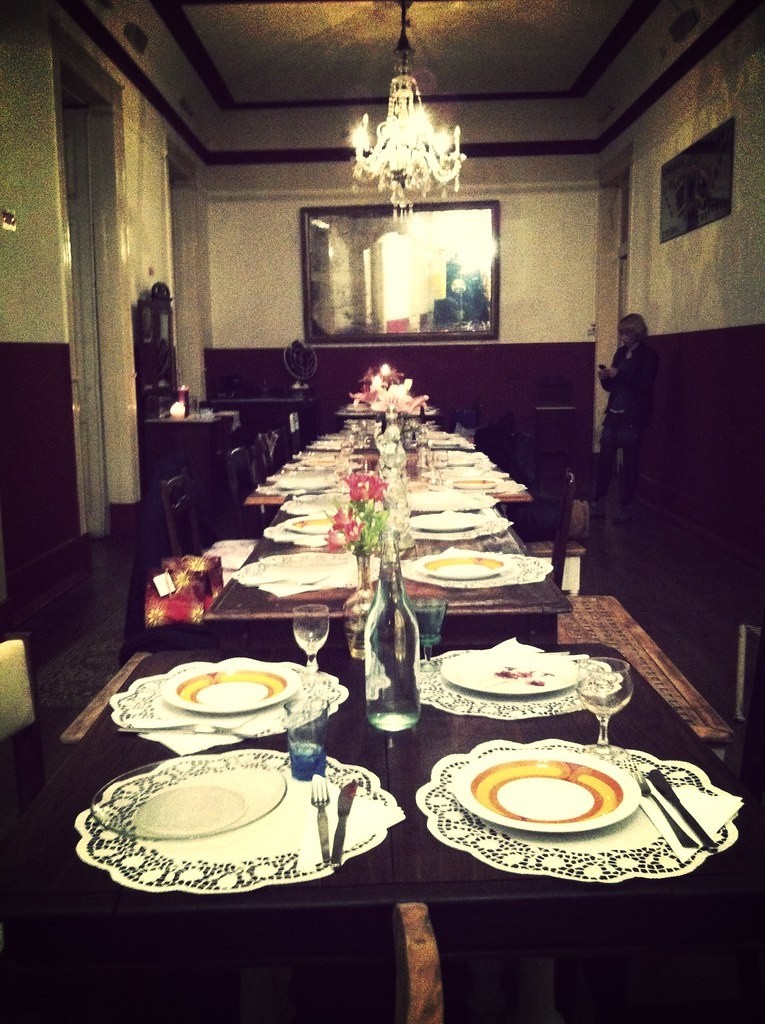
599, 365, 606, 370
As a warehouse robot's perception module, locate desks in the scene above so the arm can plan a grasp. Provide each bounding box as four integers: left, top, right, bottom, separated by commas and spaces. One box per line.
0, 639, 44, 820
0, 395, 765, 1024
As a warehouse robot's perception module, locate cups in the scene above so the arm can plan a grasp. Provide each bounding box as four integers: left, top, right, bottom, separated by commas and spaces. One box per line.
284, 697, 329, 779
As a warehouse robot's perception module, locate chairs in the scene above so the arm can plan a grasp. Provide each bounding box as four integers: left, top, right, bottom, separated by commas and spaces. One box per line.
222, 444, 270, 542
548, 447, 575, 596
159, 467, 204, 564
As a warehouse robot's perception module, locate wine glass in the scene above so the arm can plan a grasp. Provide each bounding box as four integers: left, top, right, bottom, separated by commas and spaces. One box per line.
293, 382, 436, 677
576, 656, 636, 762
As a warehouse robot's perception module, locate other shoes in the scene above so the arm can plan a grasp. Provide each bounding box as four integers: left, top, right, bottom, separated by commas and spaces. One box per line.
611, 506, 631, 524
589, 501, 605, 517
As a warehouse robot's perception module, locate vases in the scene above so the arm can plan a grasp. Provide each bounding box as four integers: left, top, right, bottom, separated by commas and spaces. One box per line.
340, 552, 375, 663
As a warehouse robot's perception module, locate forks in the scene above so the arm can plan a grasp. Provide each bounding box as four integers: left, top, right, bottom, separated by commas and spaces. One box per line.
619, 758, 700, 849
308, 776, 334, 865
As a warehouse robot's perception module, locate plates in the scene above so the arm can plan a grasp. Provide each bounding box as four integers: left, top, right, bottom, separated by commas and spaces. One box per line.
90, 427, 345, 836
400, 423, 641, 831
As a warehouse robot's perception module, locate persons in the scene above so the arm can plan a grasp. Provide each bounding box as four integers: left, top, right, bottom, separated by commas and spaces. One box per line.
584, 314, 658, 530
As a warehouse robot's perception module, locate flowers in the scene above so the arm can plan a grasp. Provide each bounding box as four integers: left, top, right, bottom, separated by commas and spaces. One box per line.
349, 359, 433, 412
327, 474, 397, 558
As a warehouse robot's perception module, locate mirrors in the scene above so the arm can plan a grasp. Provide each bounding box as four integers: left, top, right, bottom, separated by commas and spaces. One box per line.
298, 199, 500, 345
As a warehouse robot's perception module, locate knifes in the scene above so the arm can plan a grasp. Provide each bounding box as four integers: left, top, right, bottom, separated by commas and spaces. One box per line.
647, 769, 716, 847
330, 780, 359, 864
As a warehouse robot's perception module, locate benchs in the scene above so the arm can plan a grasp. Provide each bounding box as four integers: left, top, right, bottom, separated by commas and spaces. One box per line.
501, 503, 588, 597
557, 596, 735, 762
60, 541, 256, 744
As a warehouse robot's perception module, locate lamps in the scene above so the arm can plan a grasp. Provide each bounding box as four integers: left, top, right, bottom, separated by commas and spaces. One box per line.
338, 0, 466, 234
